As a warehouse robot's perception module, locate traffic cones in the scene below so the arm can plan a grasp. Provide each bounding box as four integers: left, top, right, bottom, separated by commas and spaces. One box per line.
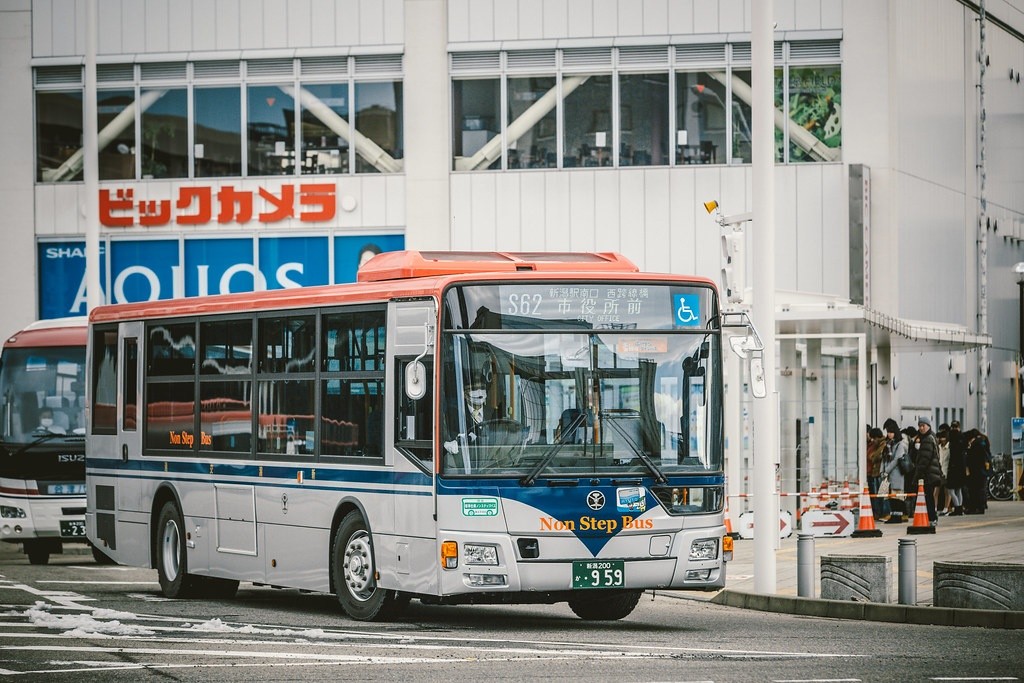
850, 487, 883, 538
908, 478, 937, 535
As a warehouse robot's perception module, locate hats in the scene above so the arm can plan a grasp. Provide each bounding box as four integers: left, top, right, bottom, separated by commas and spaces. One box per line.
940, 430, 950, 440
918, 417, 931, 427
464, 371, 486, 390
951, 420, 959, 427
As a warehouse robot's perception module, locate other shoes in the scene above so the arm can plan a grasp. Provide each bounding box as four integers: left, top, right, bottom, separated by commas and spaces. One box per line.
884, 515, 902, 523
930, 520, 937, 526
965, 505, 984, 514
939, 512, 948, 516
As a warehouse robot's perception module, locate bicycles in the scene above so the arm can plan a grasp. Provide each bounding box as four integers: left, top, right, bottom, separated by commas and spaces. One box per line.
985, 451, 1013, 500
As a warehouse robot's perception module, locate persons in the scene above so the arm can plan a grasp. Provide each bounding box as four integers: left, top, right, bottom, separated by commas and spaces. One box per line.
29, 407, 68, 437
867, 418, 992, 523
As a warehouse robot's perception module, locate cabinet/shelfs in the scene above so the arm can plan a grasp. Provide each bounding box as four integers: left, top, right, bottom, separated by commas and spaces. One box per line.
461, 130, 499, 156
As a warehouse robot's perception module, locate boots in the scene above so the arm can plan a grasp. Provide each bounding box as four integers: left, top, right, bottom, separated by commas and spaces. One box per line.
949, 506, 962, 515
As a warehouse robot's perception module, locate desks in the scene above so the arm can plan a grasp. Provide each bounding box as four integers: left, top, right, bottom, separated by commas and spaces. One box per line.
679, 145, 719, 165
255, 145, 348, 174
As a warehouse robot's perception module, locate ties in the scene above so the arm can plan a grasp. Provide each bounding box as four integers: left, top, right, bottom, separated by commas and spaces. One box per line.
473, 409, 480, 425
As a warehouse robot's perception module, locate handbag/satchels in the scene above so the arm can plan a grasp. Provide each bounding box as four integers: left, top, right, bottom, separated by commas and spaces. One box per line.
985, 452, 993, 476
895, 443, 914, 476
878, 478, 891, 495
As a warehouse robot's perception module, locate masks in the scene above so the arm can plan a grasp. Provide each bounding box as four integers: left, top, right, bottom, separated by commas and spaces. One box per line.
468, 389, 486, 405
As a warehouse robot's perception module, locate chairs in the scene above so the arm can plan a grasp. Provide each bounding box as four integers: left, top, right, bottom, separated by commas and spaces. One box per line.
41, 396, 71, 433
686, 141, 712, 165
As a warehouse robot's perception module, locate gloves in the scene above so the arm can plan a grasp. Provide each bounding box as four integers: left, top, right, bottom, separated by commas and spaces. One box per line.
880, 472, 888, 478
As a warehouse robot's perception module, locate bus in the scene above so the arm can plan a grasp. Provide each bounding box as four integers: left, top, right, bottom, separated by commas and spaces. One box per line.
0, 315, 105, 566
84, 248, 766, 623
